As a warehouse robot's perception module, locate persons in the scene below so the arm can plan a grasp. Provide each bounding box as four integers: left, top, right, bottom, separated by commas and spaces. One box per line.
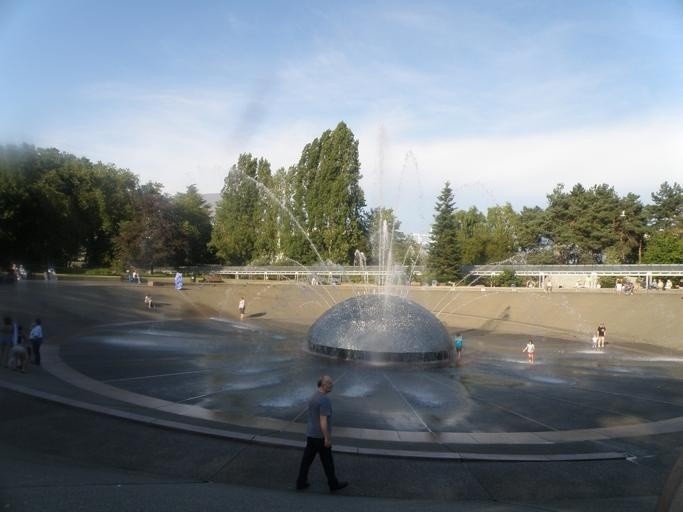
309, 273, 316, 286
68, 260, 73, 273
314, 275, 321, 286
63, 261, 68, 273
143, 292, 152, 310
452, 331, 464, 359
542, 276, 548, 294
613, 276, 672, 296
47, 263, 57, 280
237, 296, 246, 319
546, 279, 552, 294
574, 279, 582, 293
595, 322, 606, 349
520, 339, 536, 366
294, 374, 349, 494
10, 263, 21, 281
0, 315, 44, 374
123, 264, 133, 283
524, 278, 536, 288
590, 334, 597, 349
132, 269, 143, 285
18, 264, 28, 280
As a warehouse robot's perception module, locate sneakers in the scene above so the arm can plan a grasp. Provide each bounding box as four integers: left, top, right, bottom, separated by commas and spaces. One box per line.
329, 481, 348, 491
296, 483, 310, 490
